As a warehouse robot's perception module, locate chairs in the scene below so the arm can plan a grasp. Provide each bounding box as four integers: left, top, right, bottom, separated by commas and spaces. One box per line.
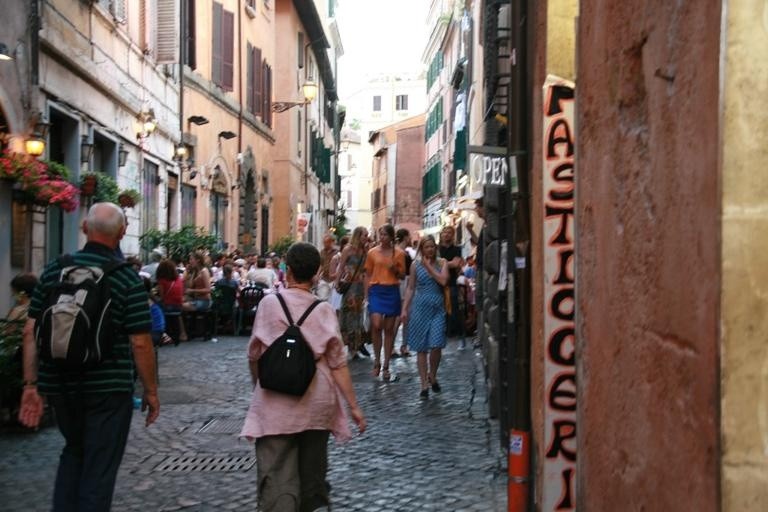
236, 286, 265, 335
186, 284, 216, 342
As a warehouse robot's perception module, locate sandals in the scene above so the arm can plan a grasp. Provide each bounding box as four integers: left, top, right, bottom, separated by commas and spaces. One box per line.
353, 343, 409, 379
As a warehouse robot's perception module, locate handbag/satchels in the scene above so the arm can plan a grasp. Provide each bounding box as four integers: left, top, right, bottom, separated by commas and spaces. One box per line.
444, 286, 453, 315
336, 279, 351, 294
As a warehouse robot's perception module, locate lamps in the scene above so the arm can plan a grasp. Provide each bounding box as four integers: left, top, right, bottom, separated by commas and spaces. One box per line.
23, 106, 52, 141
118, 142, 129, 167
129, 110, 155, 140
81, 134, 97, 164
21, 137, 46, 159
172, 143, 190, 161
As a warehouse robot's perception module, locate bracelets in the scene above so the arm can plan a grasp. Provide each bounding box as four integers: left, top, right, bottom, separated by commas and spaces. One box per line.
20, 378, 38, 387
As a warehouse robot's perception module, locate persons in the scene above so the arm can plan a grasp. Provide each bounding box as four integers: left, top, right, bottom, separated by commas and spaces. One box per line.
1, 272, 40, 401
237, 240, 369, 512
315, 225, 478, 400
13, 198, 161, 511
465, 194, 487, 349
130, 248, 287, 344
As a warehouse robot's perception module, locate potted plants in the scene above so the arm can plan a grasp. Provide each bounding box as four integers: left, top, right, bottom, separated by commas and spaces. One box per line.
117, 189, 142, 210
77, 169, 118, 204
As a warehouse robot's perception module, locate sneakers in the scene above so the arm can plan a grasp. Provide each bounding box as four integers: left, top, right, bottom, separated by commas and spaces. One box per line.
420, 389, 429, 400
432, 383, 440, 392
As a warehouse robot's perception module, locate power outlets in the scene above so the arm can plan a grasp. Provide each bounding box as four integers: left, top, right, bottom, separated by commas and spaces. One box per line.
270, 74, 318, 113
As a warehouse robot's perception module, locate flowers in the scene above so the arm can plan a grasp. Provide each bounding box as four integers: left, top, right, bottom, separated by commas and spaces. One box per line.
1, 149, 81, 213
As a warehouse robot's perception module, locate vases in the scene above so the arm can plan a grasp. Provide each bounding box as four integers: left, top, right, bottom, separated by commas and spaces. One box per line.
11, 189, 49, 214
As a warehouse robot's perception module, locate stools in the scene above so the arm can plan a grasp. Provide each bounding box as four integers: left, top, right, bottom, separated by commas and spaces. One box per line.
165, 311, 187, 347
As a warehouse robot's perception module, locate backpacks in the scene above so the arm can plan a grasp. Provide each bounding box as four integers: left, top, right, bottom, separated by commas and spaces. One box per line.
35, 254, 129, 371
258, 293, 322, 396
